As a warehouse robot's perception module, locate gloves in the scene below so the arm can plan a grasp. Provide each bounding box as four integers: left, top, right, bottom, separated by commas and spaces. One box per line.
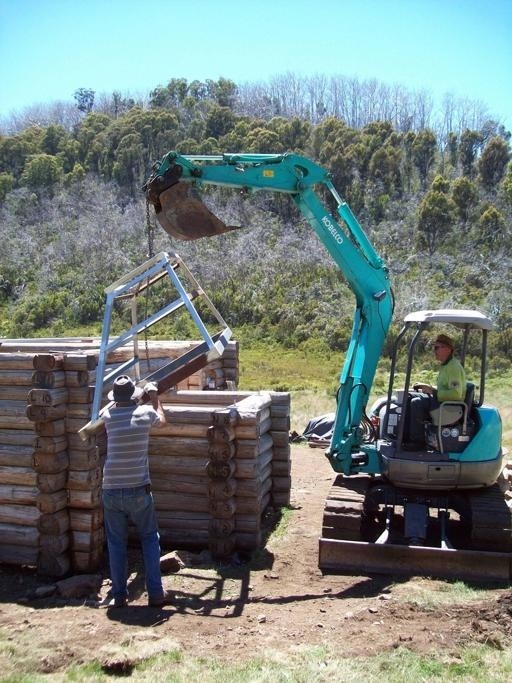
143, 381, 158, 394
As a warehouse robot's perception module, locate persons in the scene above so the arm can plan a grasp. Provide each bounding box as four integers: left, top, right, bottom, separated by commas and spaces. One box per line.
98, 374, 178, 607
403, 332, 467, 451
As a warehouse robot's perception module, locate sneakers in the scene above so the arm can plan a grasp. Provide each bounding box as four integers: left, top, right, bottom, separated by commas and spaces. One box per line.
147, 588, 170, 606
382, 433, 420, 452
113, 596, 128, 607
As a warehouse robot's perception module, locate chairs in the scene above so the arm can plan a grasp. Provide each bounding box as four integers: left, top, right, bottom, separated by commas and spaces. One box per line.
425, 383, 476, 449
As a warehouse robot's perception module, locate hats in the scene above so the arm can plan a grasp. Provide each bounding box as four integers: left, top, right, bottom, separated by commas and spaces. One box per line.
106, 374, 144, 403
430, 333, 454, 348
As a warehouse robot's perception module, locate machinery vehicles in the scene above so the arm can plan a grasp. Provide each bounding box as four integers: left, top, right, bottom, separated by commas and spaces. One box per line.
141, 151, 512, 586
